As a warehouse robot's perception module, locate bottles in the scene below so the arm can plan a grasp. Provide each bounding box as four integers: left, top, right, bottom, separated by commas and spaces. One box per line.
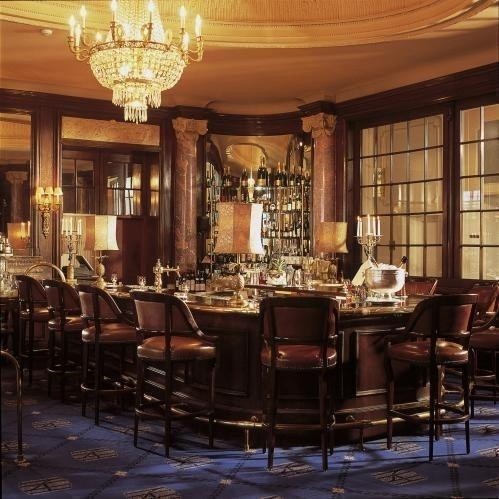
398, 256, 407, 269
242, 266, 312, 290
363, 248, 380, 268
174, 272, 207, 293
209, 160, 309, 265
0, 237, 11, 255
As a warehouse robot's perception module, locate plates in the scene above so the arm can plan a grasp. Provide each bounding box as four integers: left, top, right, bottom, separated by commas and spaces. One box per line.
106, 283, 155, 294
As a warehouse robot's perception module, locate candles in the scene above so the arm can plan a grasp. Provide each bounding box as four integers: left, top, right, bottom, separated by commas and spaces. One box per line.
21, 222, 29, 238
356, 215, 381, 238
60, 218, 81, 236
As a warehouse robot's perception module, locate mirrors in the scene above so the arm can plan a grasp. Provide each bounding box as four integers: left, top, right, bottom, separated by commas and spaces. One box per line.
0, 112, 32, 256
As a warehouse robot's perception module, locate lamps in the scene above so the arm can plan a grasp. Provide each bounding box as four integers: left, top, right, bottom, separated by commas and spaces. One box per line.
354, 212, 383, 261
35, 184, 66, 239
83, 214, 121, 286
19, 220, 32, 255
60, 216, 83, 285
211, 202, 265, 307
314, 220, 349, 260
64, 0, 206, 125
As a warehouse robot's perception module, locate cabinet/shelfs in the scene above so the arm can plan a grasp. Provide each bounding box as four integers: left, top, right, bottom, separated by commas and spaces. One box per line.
201, 183, 310, 262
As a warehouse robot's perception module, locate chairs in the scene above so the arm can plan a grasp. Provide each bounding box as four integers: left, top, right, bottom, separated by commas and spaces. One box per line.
1, 262, 499, 472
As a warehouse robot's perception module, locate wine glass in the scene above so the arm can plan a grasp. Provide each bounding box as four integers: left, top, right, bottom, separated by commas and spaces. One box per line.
111, 271, 146, 293
